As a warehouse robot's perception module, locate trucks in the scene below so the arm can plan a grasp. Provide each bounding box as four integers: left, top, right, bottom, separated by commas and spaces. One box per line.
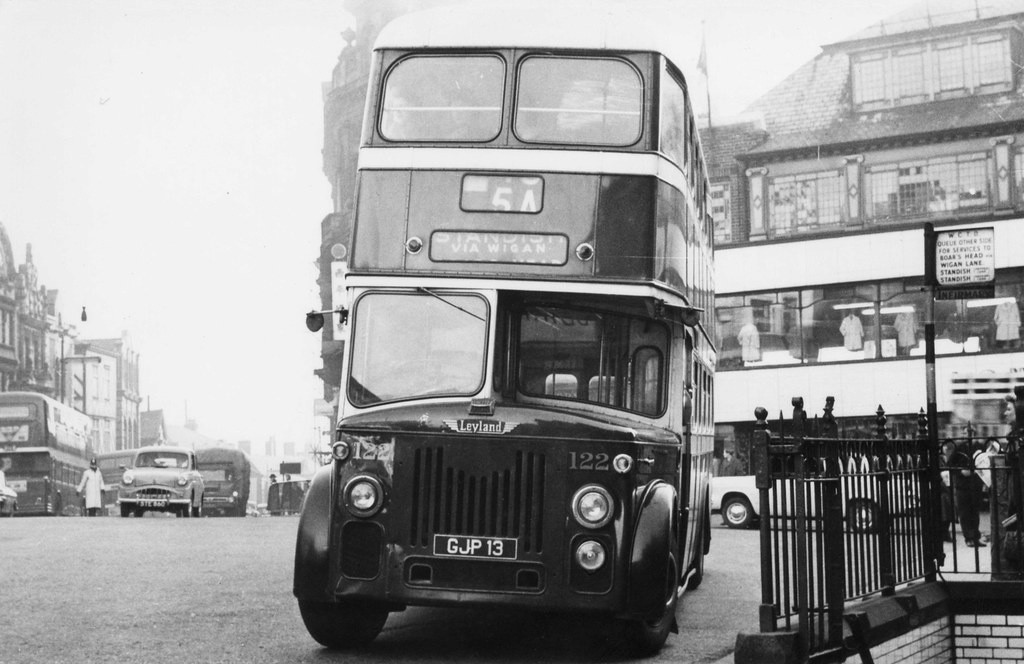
193, 447, 250, 518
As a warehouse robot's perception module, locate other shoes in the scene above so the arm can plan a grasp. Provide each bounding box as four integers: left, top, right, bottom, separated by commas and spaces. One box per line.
967, 540, 986, 548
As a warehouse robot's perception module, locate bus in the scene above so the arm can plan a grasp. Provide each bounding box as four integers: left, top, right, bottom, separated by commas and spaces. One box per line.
1, 391, 96, 518
284, 40, 723, 664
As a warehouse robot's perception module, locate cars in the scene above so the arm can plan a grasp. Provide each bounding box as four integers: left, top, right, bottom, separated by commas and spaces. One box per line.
116, 444, 203, 518
707, 456, 922, 531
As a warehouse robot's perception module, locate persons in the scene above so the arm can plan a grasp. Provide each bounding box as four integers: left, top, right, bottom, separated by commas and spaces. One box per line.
265, 472, 311, 520
76, 458, 107, 516
920, 395, 1023, 581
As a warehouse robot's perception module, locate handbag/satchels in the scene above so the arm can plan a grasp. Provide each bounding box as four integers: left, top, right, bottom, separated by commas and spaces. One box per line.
1002, 491, 1024, 562
